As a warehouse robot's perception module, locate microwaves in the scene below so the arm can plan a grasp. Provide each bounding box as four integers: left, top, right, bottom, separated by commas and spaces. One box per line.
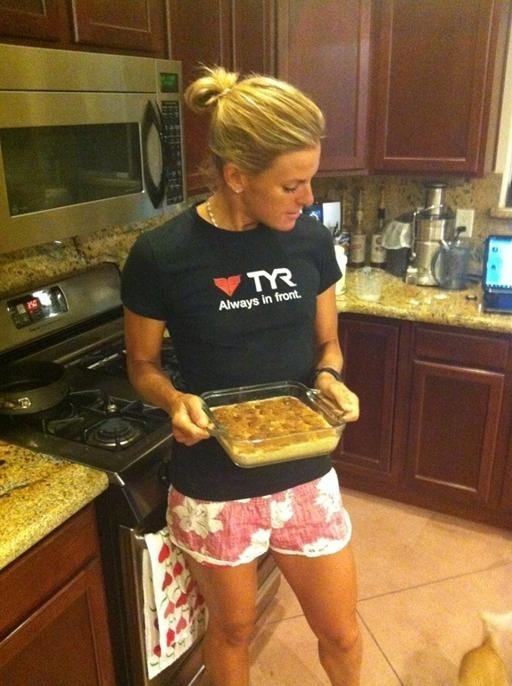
0, 43, 187, 256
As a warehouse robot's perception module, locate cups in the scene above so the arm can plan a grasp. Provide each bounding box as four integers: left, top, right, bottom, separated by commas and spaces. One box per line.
431, 240, 468, 290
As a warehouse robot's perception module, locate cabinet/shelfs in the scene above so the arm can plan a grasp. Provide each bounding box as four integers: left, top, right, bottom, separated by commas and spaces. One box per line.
0, 500, 119, 686
330, 311, 511, 533
2, 2, 230, 200
231, 2, 510, 201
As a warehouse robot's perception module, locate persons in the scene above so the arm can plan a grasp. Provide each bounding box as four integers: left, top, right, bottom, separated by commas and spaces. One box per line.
120, 61, 365, 685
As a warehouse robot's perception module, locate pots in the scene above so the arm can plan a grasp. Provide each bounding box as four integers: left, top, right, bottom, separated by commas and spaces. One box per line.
1, 361, 69, 415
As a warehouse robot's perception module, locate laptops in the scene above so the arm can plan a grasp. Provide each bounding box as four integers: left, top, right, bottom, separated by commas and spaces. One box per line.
482, 236, 512, 314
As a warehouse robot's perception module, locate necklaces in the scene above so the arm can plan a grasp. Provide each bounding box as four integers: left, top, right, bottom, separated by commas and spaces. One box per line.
206, 200, 220, 228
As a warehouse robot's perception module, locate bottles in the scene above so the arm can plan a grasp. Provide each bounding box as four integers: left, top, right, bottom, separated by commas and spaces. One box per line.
350, 188, 388, 269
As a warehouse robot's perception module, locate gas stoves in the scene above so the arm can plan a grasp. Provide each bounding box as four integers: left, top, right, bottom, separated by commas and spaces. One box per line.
20, 329, 191, 529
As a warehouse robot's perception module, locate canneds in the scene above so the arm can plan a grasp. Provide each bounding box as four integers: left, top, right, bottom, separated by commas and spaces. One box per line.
339, 232, 350, 265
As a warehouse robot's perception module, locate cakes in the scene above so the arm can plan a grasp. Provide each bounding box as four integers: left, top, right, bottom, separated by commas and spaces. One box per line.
209, 395, 340, 465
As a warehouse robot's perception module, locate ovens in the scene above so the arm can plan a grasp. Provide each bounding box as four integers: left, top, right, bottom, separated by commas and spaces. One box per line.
118, 524, 281, 686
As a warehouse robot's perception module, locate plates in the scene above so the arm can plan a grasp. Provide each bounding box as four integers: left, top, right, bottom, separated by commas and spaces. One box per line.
199, 379, 347, 469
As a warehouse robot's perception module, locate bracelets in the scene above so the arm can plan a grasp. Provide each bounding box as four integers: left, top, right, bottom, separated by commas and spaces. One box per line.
313, 367, 341, 384
